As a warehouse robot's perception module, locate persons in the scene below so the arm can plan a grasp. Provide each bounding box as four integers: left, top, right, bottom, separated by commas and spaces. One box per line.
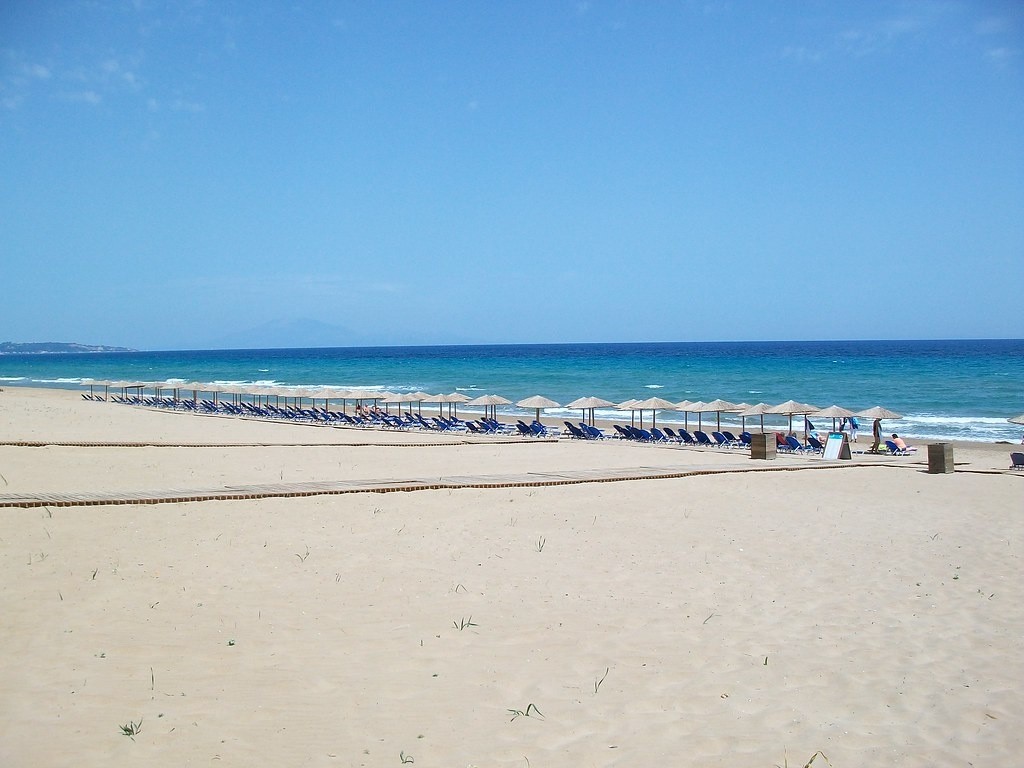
872, 418, 882, 453
356, 399, 381, 415
848, 417, 858, 443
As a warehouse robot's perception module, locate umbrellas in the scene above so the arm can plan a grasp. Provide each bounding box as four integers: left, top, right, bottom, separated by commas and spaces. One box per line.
515, 395, 560, 422
1007, 414, 1024, 424
81, 380, 903, 449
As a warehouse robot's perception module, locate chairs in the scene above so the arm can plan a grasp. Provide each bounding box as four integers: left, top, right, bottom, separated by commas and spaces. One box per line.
73, 393, 516, 435
613, 424, 825, 457
864, 440, 919, 457
1009, 452, 1024, 470
562, 421, 613, 442
516, 419, 561, 438
